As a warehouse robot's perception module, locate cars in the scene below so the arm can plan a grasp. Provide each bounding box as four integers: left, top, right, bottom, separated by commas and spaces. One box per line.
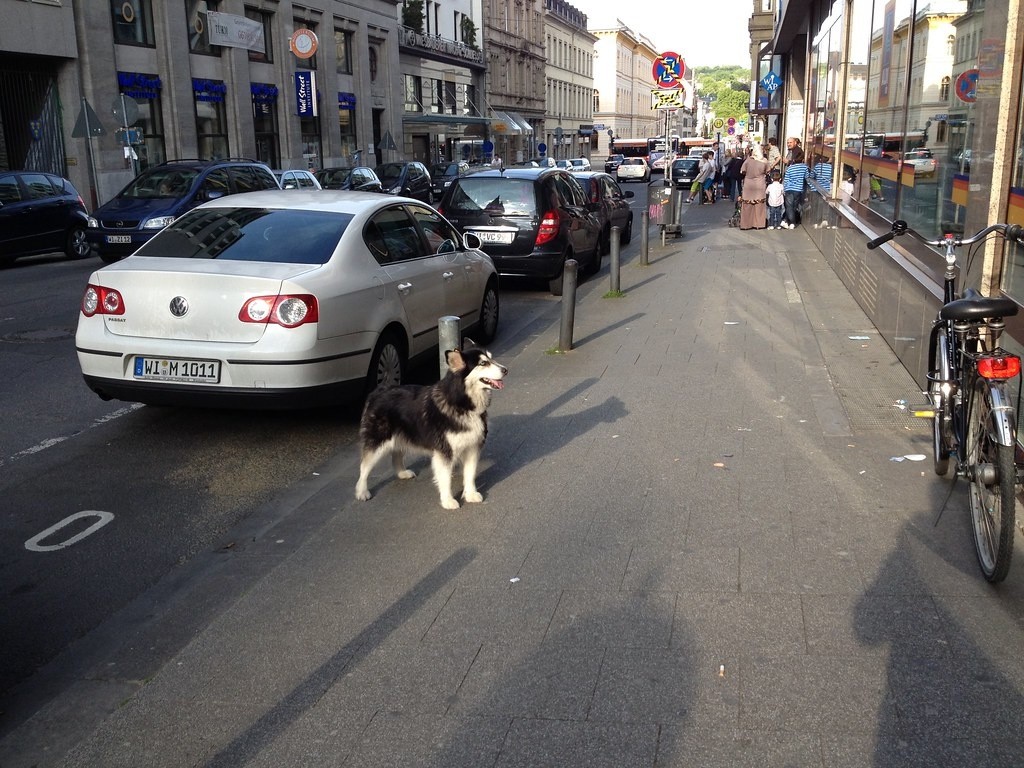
565, 170, 635, 258
604, 154, 624, 172
437, 166, 600, 281
958, 149, 995, 173
0, 171, 92, 265
898, 147, 936, 176
663, 147, 716, 191
76, 189, 502, 413
87, 157, 281, 266
272, 158, 590, 204
617, 156, 651, 182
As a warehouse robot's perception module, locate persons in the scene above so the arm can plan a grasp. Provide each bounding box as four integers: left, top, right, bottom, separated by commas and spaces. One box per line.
768, 138, 781, 173
783, 137, 804, 167
492, 154, 503, 165
810, 156, 832, 229
740, 146, 770, 230
684, 142, 752, 204
780, 151, 809, 229
832, 171, 854, 229
469, 154, 478, 164
580, 153, 585, 158
766, 173, 784, 231
846, 137, 886, 204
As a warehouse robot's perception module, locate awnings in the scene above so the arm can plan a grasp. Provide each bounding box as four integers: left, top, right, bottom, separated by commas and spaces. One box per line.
491, 111, 533, 136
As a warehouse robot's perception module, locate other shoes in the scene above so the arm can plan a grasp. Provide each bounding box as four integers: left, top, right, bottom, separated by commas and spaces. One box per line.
690, 197, 693, 201
703, 200, 712, 204
683, 200, 690, 203
780, 220, 789, 229
789, 224, 795, 230
777, 227, 781, 229
721, 197, 729, 200
766, 226, 774, 230
872, 196, 878, 200
879, 197, 889, 203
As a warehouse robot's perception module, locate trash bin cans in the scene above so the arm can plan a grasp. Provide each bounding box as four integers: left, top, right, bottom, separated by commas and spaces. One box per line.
647, 178, 676, 225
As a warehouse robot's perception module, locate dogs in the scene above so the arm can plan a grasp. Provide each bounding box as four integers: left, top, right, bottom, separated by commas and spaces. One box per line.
354, 337, 508, 511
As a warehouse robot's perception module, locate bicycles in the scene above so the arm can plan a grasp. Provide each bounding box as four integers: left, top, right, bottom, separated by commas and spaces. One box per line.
867, 218, 1024, 585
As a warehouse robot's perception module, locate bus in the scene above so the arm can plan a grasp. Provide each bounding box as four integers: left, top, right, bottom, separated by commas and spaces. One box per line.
648, 136, 682, 172
821, 130, 927, 164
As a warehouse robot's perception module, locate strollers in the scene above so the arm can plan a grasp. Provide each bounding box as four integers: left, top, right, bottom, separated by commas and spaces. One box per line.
727, 189, 743, 228
